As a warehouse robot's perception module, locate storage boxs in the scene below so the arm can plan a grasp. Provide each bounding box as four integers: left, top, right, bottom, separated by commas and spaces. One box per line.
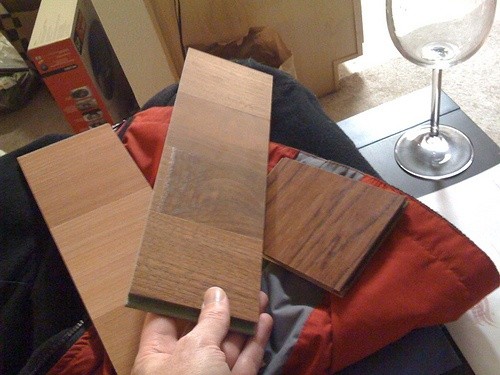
27, 0, 140, 134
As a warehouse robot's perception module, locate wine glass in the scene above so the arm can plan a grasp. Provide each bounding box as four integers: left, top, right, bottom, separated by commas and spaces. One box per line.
385, 0, 498, 180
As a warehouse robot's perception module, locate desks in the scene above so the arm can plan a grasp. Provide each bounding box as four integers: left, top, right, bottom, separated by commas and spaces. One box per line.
336, 85, 500, 198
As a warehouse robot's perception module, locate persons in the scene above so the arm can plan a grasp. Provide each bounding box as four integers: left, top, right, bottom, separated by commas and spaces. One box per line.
130, 287, 273, 375
